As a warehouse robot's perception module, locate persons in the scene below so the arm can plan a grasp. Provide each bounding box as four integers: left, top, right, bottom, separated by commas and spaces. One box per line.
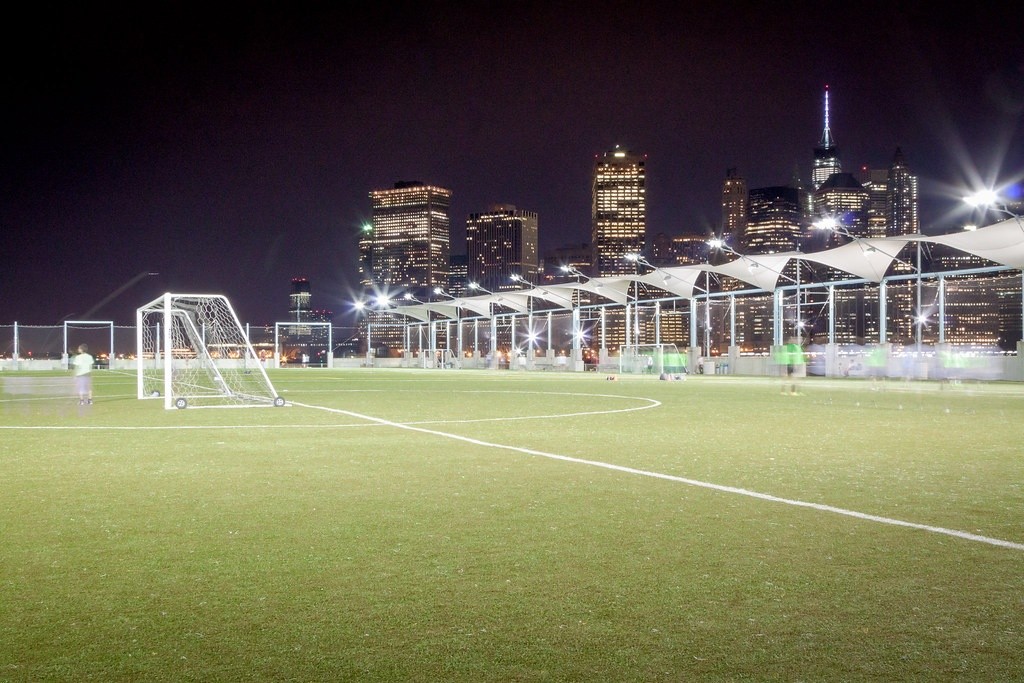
302, 353, 310, 368
647, 355, 653, 374
261, 349, 268, 366
74, 343, 95, 406
26, 352, 33, 360
806, 337, 825, 376
781, 333, 804, 396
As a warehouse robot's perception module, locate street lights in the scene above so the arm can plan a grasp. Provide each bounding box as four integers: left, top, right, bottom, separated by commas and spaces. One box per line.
622, 252, 709, 376
967, 189, 1024, 234
706, 237, 803, 379
434, 289, 462, 370
354, 301, 405, 370
562, 264, 638, 373
818, 215, 923, 380
510, 274, 580, 369
468, 282, 533, 370
402, 292, 432, 371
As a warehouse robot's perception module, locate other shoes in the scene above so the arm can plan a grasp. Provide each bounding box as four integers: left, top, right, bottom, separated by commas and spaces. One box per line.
79, 400, 84, 405
86, 398, 93, 404
